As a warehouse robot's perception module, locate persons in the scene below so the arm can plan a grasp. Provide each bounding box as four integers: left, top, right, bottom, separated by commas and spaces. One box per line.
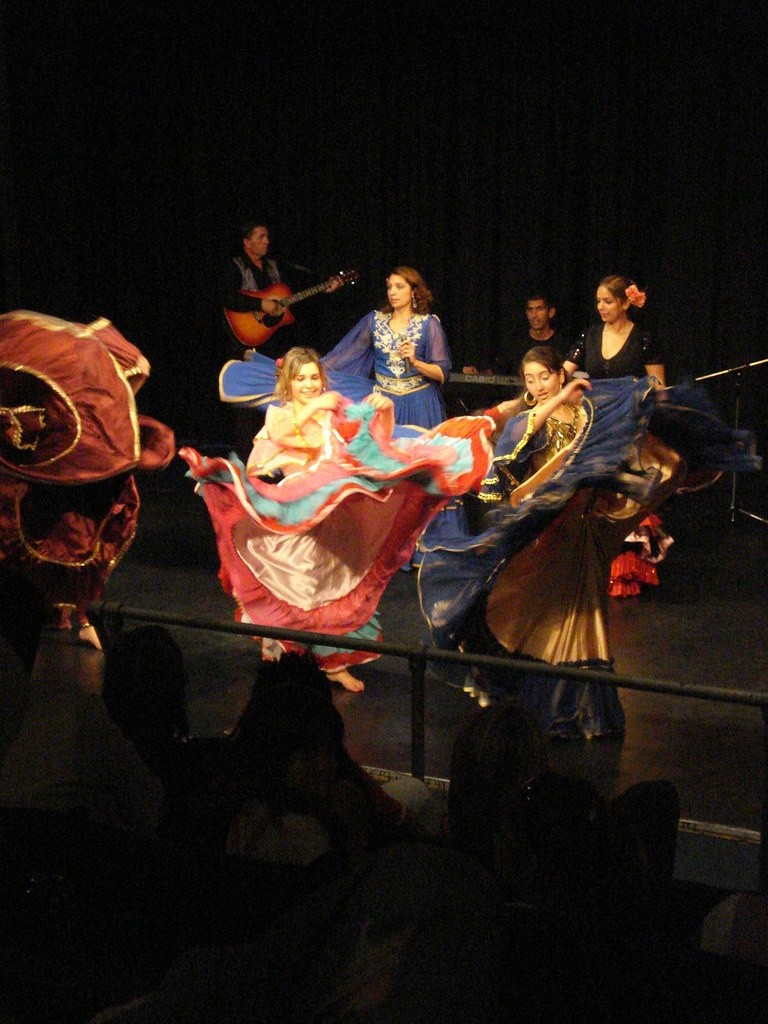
0, 623, 704, 941
0, 308, 177, 629
417, 345, 763, 741
178, 347, 498, 692
217, 221, 345, 360
218, 266, 470, 571
491, 293, 577, 400
576, 274, 675, 598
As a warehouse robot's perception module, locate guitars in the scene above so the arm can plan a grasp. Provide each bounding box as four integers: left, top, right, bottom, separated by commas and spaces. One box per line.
224, 269, 360, 346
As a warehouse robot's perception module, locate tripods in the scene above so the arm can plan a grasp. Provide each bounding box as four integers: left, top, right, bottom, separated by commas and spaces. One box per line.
665, 359, 768, 525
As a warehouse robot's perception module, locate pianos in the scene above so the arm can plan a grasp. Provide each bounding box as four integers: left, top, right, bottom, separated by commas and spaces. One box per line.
447, 371, 525, 389
